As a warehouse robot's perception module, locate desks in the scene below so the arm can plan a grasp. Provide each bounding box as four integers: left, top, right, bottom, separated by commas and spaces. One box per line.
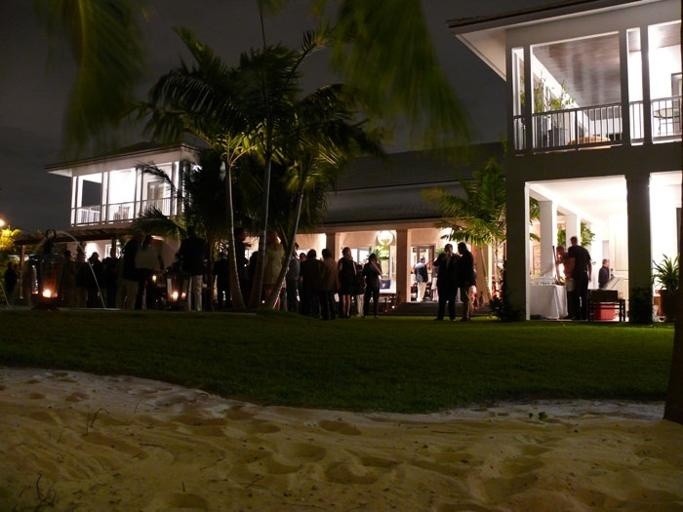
530, 283, 582, 319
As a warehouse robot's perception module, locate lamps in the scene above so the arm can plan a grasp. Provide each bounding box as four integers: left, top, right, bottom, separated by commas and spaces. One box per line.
378, 230, 394, 246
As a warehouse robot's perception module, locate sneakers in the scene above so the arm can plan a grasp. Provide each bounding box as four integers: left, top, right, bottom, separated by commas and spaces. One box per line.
565, 310, 587, 321
433, 315, 471, 321
290, 311, 379, 319
4, 298, 148, 310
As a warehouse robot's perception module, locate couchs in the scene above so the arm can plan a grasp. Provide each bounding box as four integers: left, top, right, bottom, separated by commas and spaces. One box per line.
567, 133, 610, 146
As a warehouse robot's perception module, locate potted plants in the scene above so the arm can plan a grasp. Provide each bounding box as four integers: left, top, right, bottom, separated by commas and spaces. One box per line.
650, 252, 682, 322
521, 70, 578, 146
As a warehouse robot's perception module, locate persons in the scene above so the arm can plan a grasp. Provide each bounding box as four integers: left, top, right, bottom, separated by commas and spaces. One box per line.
596, 258, 612, 290
452, 241, 476, 321
2, 261, 26, 306
564, 236, 592, 319
431, 243, 460, 321
411, 255, 441, 305
552, 245, 578, 320
51, 219, 384, 322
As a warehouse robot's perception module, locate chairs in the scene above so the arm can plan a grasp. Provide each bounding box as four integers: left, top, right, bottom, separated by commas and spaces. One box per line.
581, 289, 626, 323
